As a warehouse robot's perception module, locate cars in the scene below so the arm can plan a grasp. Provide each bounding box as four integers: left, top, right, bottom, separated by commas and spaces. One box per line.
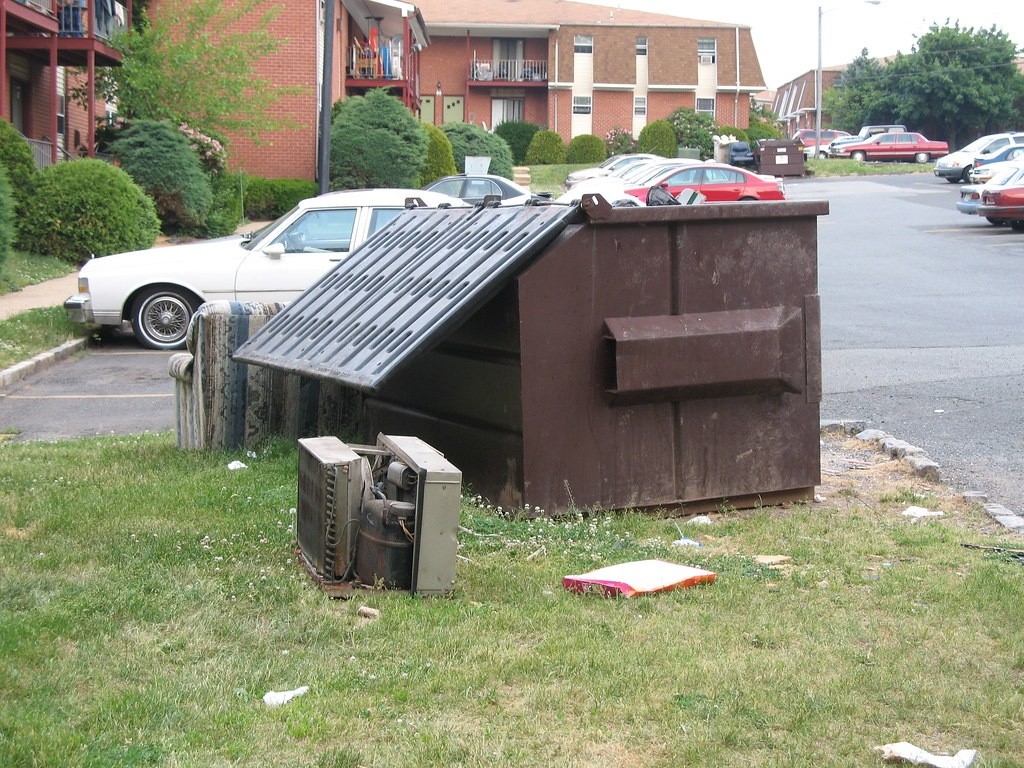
728, 141, 754, 166
555, 153, 787, 208
419, 173, 555, 206
829, 132, 949, 164
61, 187, 475, 352
791, 124, 1024, 227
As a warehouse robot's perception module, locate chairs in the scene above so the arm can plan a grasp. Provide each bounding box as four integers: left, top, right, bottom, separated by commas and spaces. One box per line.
727, 143, 754, 168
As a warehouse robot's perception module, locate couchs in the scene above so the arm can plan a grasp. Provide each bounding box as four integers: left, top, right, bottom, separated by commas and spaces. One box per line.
168, 300, 368, 453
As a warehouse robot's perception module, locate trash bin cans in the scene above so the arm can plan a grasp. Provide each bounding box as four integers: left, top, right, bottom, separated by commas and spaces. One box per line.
230, 195, 832, 519
713, 141, 739, 165
752, 138, 806, 177
